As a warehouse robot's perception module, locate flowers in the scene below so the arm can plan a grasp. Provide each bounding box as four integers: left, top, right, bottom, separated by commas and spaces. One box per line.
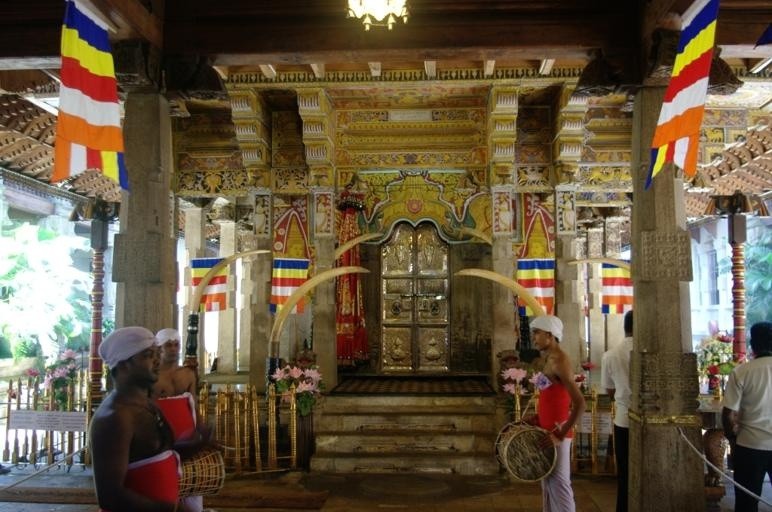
494, 362, 552, 415
269, 361, 328, 417
6, 347, 83, 411
693, 320, 752, 403
573, 357, 599, 392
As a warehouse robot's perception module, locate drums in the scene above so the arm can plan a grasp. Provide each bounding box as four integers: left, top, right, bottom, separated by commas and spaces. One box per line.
494, 420, 558, 483
177, 446, 226, 500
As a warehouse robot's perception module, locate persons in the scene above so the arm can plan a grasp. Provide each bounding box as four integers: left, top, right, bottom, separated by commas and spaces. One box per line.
151, 325, 210, 512
720, 320, 771, 512
528, 315, 586, 512
87, 326, 184, 512
599, 311, 634, 512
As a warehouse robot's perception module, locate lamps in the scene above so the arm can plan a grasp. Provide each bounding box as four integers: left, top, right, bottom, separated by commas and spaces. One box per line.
343, 0, 415, 34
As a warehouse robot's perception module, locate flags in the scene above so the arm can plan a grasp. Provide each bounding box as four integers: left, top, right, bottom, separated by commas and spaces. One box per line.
267, 257, 310, 316
643, 0, 721, 192
516, 256, 557, 320
190, 257, 228, 312
48, 0, 131, 191
600, 259, 633, 315
